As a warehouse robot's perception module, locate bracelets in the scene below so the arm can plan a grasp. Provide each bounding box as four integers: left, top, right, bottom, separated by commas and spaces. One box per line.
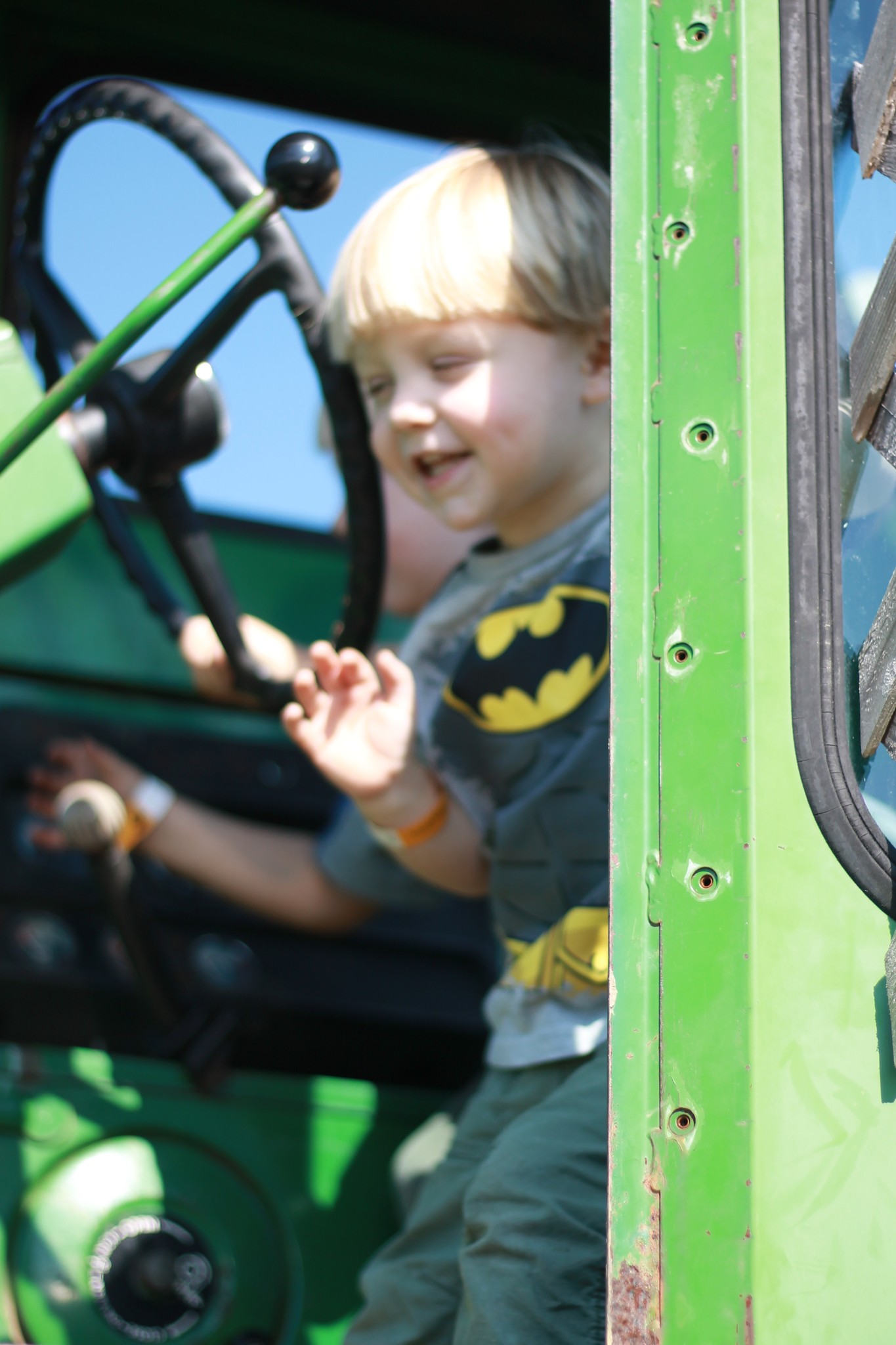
114, 776, 175, 851
368, 769, 451, 851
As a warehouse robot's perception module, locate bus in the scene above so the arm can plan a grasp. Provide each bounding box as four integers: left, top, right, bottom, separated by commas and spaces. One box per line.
0, 2, 896, 1345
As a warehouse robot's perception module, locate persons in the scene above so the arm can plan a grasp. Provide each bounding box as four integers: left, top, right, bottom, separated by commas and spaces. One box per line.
27, 141, 610, 1345
